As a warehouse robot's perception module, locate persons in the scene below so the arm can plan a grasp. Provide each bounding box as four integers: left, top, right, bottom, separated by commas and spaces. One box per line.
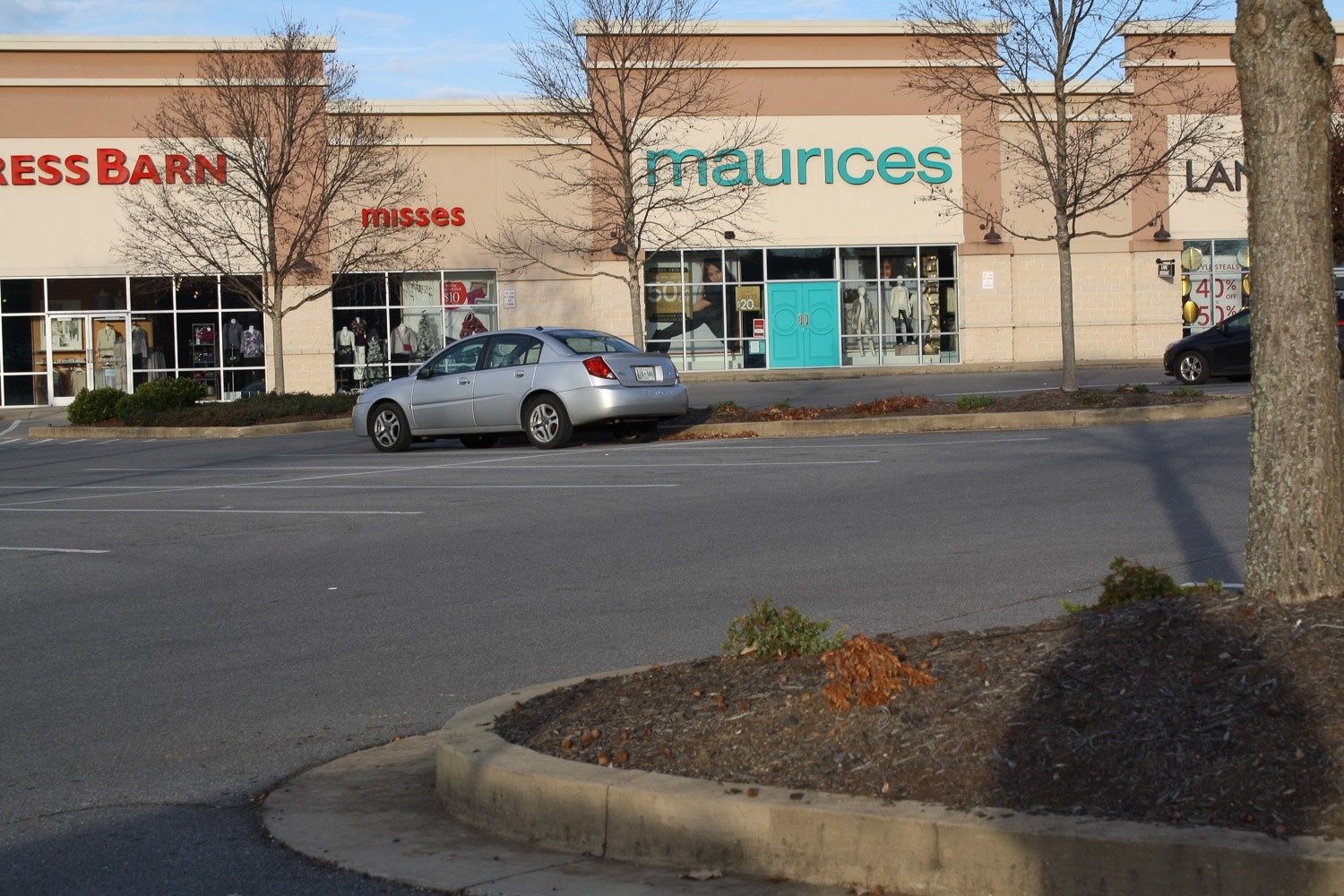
349, 317, 367, 380
335, 326, 355, 369
222, 318, 242, 351
389, 323, 417, 377
890, 275, 915, 345
858, 256, 913, 348
852, 286, 877, 357
240, 325, 264, 358
909, 283, 933, 346
647, 259, 741, 352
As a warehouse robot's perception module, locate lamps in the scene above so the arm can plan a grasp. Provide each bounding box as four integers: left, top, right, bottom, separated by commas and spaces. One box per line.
1147, 211, 1172, 239
979, 213, 1002, 240
610, 223, 629, 256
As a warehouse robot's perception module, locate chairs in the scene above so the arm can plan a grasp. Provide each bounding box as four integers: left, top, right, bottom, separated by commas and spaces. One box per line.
496, 354, 521, 367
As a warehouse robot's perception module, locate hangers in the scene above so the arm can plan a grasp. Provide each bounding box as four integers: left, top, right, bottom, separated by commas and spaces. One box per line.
44, 364, 86, 373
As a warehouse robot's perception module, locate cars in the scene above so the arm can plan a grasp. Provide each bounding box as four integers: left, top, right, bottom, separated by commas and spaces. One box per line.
348, 324, 691, 451
1161, 295, 1344, 385
